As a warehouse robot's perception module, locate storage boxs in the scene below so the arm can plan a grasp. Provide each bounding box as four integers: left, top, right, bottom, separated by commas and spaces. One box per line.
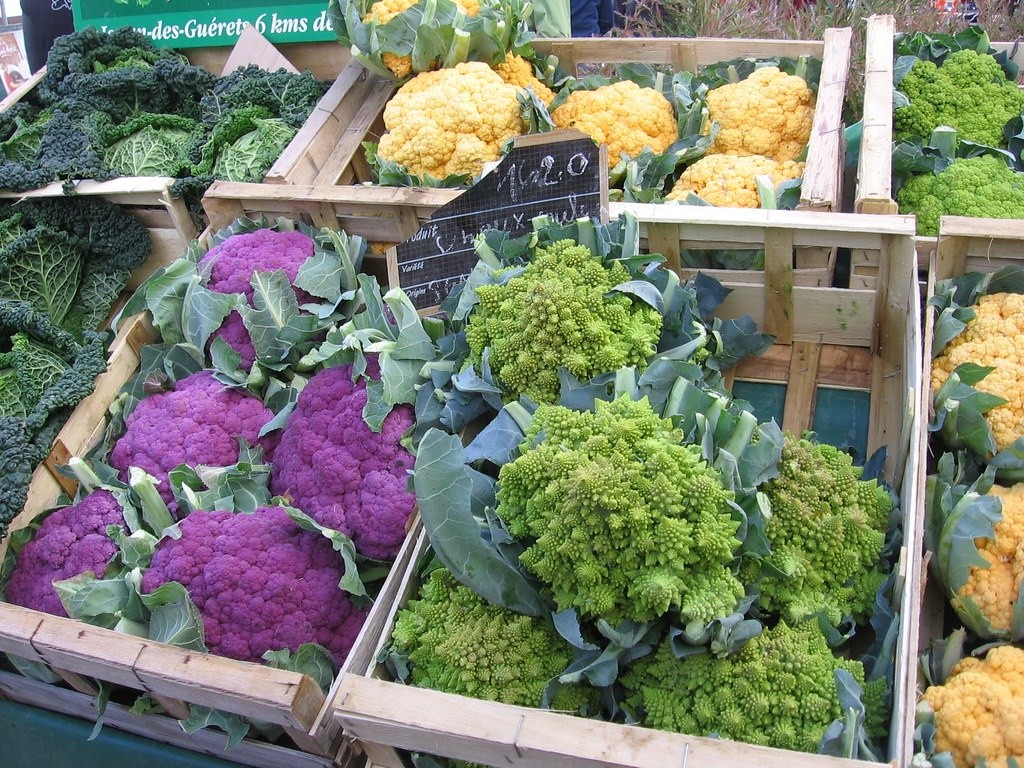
0, 14, 1024, 768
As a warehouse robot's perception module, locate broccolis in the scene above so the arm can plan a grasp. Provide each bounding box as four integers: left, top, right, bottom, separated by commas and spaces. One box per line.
0, 0, 1024, 768
